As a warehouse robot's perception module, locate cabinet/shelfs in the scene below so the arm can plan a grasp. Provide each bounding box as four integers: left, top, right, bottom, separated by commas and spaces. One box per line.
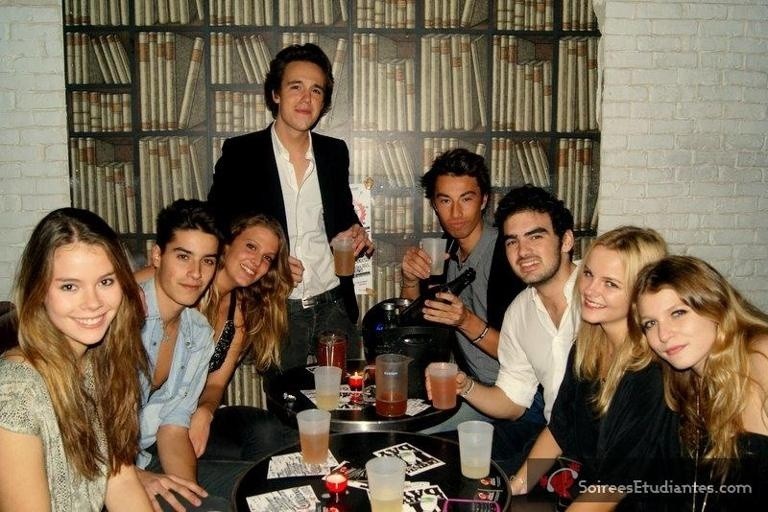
60, 0, 606, 333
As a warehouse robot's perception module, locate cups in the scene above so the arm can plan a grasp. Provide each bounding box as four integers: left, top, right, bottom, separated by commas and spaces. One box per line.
315, 330, 346, 376
427, 361, 460, 409
332, 237, 351, 275
365, 455, 404, 512
312, 365, 343, 411
315, 445, 333, 454
294, 410, 330, 461
417, 237, 447, 277
457, 420, 495, 479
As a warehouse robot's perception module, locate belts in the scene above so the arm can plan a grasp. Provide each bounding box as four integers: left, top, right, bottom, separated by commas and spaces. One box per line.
287, 286, 340, 313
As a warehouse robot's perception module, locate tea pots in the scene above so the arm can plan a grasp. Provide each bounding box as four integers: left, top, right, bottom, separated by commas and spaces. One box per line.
361, 353, 415, 417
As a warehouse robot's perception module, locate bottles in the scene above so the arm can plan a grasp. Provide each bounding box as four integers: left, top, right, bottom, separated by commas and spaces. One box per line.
402, 268, 475, 325
324, 474, 351, 512
